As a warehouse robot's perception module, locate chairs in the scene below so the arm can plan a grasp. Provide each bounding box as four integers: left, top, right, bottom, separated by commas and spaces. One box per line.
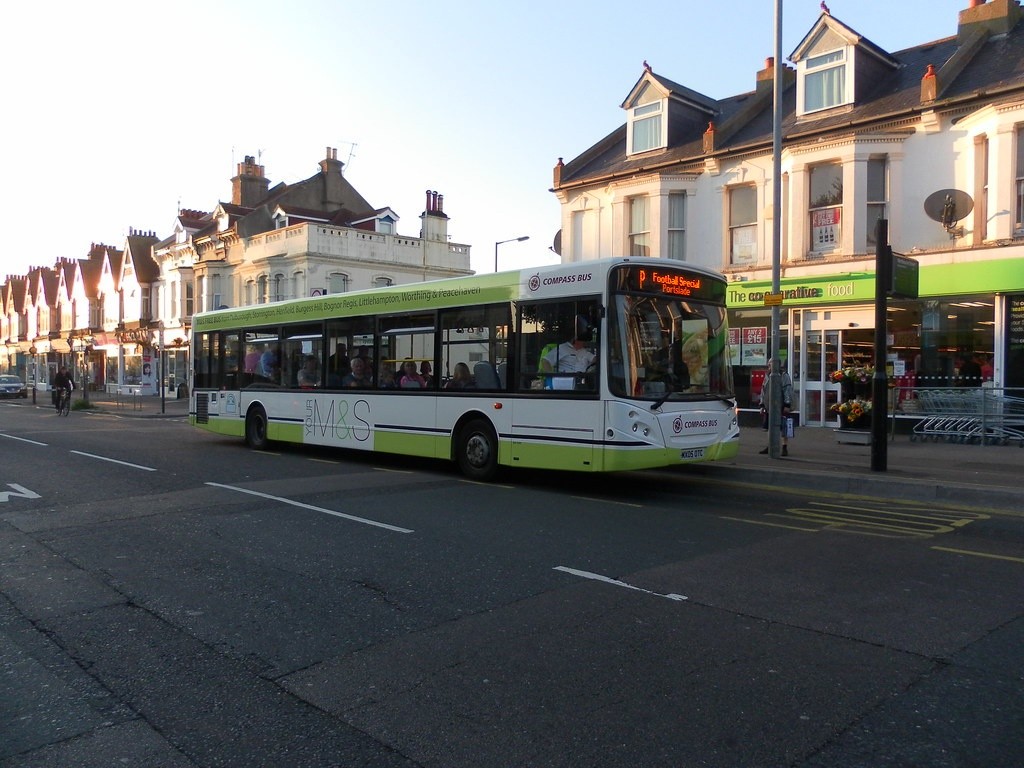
537, 342, 560, 380
498, 361, 506, 390
474, 360, 500, 389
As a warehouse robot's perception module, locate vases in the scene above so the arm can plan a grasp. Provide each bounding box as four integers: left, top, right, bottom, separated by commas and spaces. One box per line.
833, 429, 871, 445
842, 381, 873, 394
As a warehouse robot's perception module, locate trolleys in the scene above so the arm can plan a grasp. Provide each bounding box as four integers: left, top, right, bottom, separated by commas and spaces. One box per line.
910, 390, 1024, 448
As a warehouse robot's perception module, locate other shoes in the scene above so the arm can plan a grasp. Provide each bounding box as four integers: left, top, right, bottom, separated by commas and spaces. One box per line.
757, 446, 769, 455
781, 444, 788, 456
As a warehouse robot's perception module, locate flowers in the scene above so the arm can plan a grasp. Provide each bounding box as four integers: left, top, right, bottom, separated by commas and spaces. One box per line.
830, 397, 873, 423
828, 362, 875, 384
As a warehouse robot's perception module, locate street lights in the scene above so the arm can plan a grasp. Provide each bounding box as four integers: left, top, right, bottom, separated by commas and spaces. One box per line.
495, 236, 530, 272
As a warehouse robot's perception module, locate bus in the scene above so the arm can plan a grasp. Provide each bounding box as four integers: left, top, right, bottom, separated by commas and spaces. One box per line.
189, 255, 740, 482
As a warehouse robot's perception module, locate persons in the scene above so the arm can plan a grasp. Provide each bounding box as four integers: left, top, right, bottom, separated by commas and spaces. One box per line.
245, 343, 278, 375
380, 357, 433, 389
759, 358, 792, 456
915, 336, 994, 390
142, 364, 151, 385
542, 331, 596, 372
329, 343, 373, 386
226, 341, 239, 372
446, 362, 475, 389
651, 333, 690, 392
55, 367, 76, 413
289, 341, 321, 389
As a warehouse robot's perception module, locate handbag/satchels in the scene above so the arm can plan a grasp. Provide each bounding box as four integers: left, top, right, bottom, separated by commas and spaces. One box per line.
790, 391, 797, 412
782, 415, 795, 438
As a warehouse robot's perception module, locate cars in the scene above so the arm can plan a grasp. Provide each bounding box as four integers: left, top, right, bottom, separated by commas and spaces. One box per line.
0, 375, 28, 398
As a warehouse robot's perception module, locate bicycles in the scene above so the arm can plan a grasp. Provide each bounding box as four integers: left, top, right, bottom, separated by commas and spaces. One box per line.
56, 386, 73, 417
